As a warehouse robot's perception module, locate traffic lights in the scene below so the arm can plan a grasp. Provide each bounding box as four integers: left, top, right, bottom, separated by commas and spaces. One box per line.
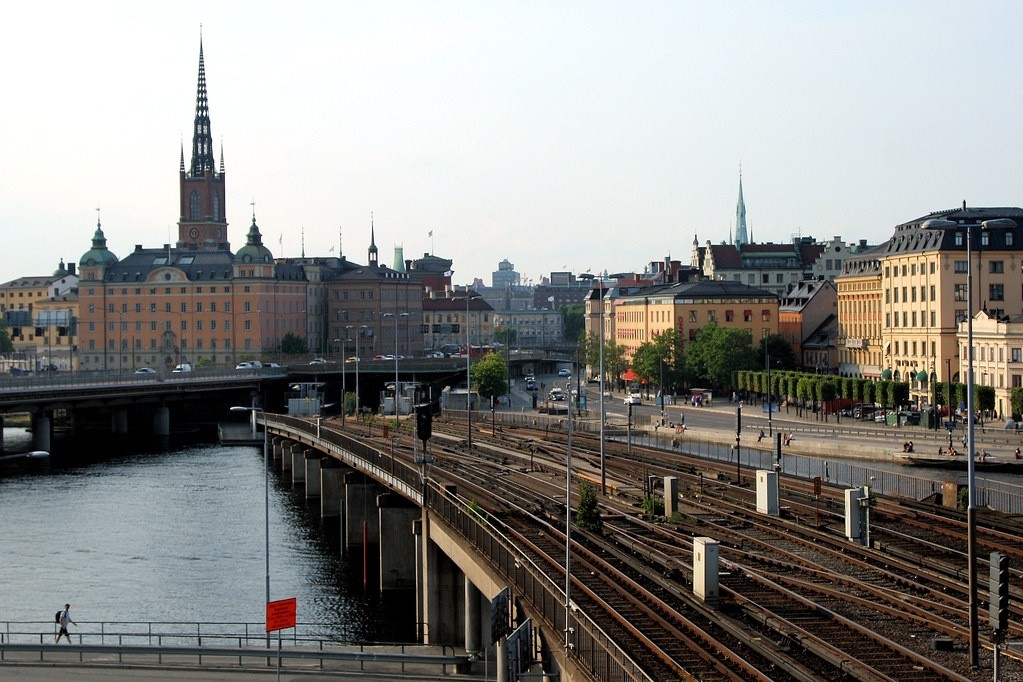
641, 380, 646, 385
417, 401, 432, 441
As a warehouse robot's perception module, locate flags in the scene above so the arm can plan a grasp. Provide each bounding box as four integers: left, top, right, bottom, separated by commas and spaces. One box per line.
428, 230, 432, 237
279, 234, 282, 244
329, 246, 334, 252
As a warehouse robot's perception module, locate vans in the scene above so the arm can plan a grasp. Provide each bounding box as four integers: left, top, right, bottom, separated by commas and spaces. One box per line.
630, 384, 640, 393
171, 363, 191, 373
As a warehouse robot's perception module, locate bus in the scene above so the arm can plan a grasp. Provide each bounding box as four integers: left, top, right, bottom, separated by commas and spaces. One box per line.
459, 345, 497, 358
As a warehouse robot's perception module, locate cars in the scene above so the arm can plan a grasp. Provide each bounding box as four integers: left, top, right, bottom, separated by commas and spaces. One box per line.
524, 374, 538, 390
373, 355, 405, 360
624, 396, 641, 405
837, 404, 980, 426
345, 357, 360, 363
558, 368, 572, 376
309, 358, 326, 365
135, 368, 156, 374
236, 361, 280, 370
548, 387, 566, 400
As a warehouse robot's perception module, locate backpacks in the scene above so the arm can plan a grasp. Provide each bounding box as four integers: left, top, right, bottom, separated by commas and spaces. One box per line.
55, 611, 67, 623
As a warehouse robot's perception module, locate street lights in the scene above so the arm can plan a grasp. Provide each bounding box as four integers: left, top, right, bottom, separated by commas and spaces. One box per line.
452, 296, 484, 456
260, 310, 306, 365
657, 351, 667, 426
304, 312, 340, 364
20, 305, 80, 378
920, 218, 1020, 671
383, 312, 411, 430
153, 308, 208, 379
346, 325, 367, 424
209, 310, 260, 370
88, 307, 150, 380
577, 272, 625, 500
232, 406, 273, 670
764, 328, 773, 436
334, 339, 352, 428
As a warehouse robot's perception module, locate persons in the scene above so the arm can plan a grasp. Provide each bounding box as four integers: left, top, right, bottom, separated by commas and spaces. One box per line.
903, 441, 914, 453
1015, 421, 1019, 435
56, 603, 77, 644
757, 430, 764, 442
961, 434, 968, 451
684, 393, 704, 407
978, 449, 986, 462
938, 446, 957, 456
654, 419, 687, 434
782, 432, 795, 446
1013, 447, 1020, 461
